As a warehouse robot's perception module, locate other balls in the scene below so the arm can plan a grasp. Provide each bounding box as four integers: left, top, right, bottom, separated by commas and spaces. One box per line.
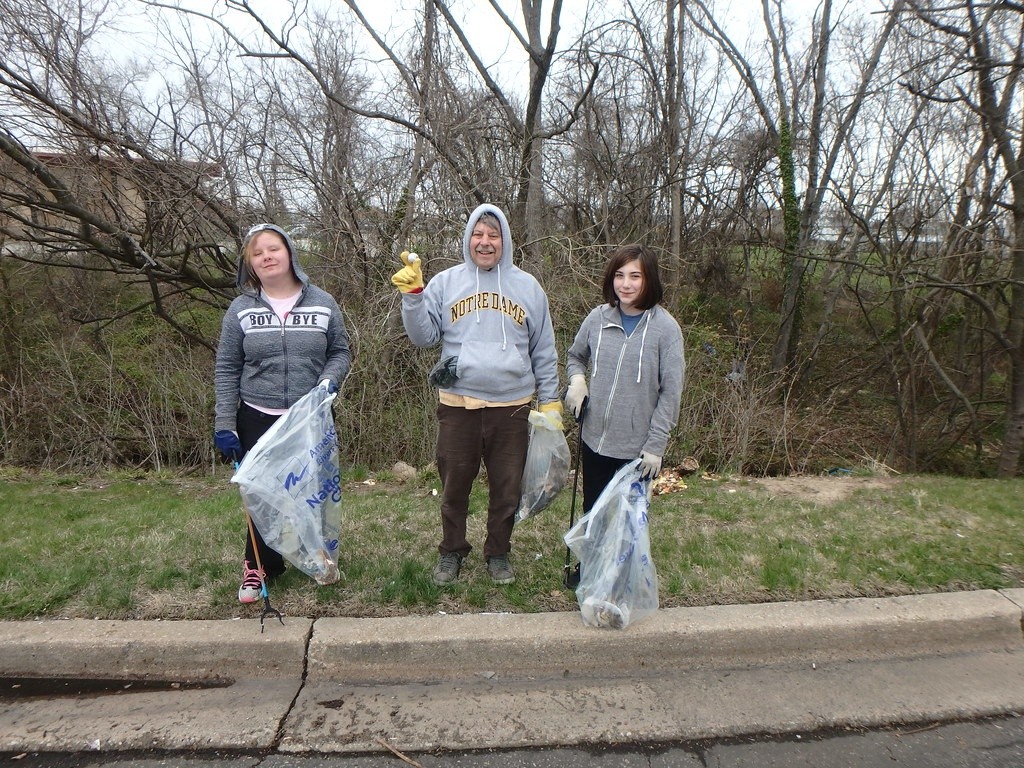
408, 253, 419, 264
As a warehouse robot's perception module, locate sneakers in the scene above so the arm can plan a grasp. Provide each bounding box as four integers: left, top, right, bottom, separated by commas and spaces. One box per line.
432, 552, 462, 587
238, 560, 266, 603
486, 554, 516, 585
566, 562, 581, 589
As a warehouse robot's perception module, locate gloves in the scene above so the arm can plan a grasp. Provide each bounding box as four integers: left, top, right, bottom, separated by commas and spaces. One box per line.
213, 430, 242, 458
390, 251, 424, 294
538, 399, 564, 430
564, 374, 589, 423
637, 449, 662, 482
314, 378, 339, 417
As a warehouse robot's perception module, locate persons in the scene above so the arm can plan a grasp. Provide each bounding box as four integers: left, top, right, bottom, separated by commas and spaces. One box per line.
390, 201, 564, 585
564, 243, 687, 597
213, 223, 354, 605
